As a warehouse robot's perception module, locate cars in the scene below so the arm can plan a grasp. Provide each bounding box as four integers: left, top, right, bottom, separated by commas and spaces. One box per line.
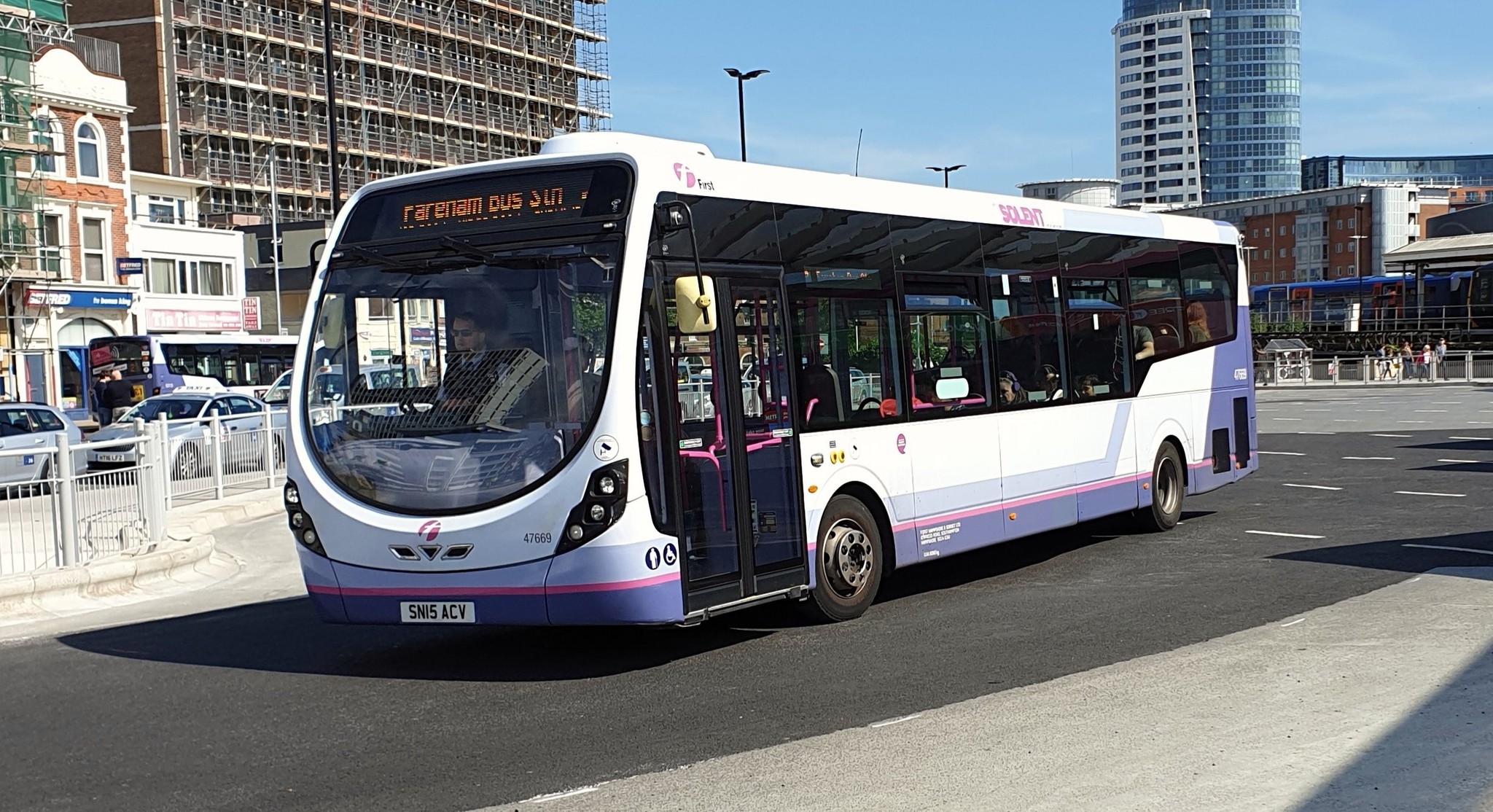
81, 385, 288, 485
262, 367, 293, 468
313, 362, 424, 402
0, 400, 89, 498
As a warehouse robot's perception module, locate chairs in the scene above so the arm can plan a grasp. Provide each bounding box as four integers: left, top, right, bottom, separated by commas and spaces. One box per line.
377, 375, 403, 388
229, 372, 275, 386
14, 419, 29, 429
854, 322, 1180, 417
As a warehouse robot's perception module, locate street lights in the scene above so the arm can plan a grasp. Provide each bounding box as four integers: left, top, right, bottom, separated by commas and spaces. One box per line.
269, 143, 286, 335
723, 67, 771, 163
925, 165, 967, 188
1353, 205, 1364, 331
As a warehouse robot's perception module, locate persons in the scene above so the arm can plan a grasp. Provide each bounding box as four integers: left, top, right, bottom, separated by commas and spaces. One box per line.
1376, 338, 1450, 382
434, 310, 510, 407
95, 369, 135, 428
1252, 339, 1268, 386
930, 302, 1210, 414
524, 334, 602, 420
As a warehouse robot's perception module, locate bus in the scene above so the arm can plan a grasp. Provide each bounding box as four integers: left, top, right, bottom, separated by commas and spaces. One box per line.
87, 334, 300, 426
1249, 270, 1473, 333
284, 131, 1259, 633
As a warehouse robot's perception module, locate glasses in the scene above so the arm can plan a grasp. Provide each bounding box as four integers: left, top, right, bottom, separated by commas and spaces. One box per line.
450, 329, 473, 337
